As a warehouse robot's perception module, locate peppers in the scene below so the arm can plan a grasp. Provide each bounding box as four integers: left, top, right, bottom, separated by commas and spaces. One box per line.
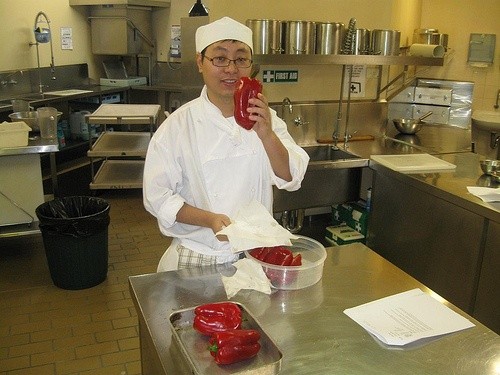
233, 70, 262, 130
193, 302, 247, 336
250, 246, 302, 285
206, 330, 260, 365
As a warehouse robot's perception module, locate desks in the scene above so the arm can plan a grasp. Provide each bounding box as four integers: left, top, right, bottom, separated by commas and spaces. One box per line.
129, 242, 500, 375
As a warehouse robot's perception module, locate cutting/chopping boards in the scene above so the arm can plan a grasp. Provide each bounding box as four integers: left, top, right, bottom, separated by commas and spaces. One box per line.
370, 153, 457, 172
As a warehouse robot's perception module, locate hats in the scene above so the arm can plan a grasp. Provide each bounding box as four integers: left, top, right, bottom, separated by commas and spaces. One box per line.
195, 16, 254, 56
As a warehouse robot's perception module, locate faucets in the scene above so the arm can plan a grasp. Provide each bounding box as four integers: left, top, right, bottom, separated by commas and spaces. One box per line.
282, 97, 293, 123
34, 10, 57, 93
494, 89, 500, 108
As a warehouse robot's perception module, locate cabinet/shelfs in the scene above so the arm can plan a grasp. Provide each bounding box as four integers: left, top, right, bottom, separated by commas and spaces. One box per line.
88, 104, 164, 189
369, 156, 500, 336
68, 0, 171, 57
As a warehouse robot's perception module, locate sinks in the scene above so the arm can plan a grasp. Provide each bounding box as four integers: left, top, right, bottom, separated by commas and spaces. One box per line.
0, 99, 36, 105
43, 89, 94, 96
471, 110, 500, 132
272, 144, 363, 212
23, 94, 62, 100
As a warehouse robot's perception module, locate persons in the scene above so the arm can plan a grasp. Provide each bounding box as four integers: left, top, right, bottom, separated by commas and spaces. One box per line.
143, 17, 309, 274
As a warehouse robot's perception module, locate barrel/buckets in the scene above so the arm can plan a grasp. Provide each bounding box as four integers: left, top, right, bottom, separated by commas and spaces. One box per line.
413, 28, 448, 54
58, 110, 101, 148
246, 17, 402, 56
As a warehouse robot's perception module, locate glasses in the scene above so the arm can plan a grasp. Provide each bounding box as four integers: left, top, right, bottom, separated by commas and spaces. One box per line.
203, 55, 253, 68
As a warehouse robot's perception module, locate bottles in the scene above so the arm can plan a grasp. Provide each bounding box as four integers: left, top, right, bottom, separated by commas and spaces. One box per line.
366, 185, 372, 212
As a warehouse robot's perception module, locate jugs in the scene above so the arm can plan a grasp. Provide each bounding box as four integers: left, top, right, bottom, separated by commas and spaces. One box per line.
10, 99, 35, 118
37, 107, 58, 139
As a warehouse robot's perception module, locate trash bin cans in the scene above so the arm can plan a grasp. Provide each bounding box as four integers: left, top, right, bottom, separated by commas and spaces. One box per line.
36, 197, 111, 291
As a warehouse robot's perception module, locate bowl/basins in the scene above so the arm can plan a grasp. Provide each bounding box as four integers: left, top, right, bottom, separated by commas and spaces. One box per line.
480, 159, 500, 174
9, 111, 63, 131
392, 118, 429, 135
243, 235, 328, 290
